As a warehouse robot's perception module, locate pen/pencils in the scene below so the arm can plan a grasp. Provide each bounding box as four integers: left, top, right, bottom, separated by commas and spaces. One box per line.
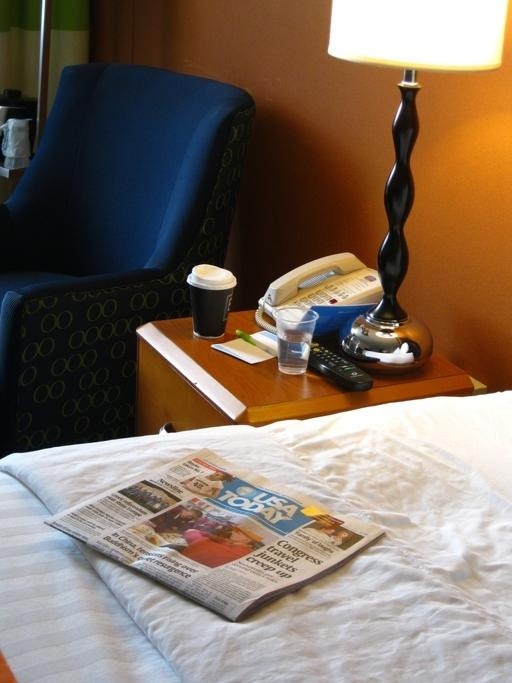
235, 329, 278, 356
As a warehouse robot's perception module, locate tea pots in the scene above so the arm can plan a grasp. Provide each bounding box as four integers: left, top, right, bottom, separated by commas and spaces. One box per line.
0, 116, 33, 170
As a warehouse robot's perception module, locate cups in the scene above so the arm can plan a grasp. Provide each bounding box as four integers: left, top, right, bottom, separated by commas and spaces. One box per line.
186, 263, 237, 340
272, 305, 318, 376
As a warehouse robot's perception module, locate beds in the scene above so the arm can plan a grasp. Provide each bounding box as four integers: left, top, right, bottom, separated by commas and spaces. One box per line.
0, 387, 511, 682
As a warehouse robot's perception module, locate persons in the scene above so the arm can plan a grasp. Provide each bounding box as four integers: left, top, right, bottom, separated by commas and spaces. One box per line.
183, 471, 231, 498
304, 526, 353, 547
149, 505, 263, 568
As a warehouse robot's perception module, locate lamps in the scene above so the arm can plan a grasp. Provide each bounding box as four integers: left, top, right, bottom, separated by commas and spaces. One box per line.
326, 0, 507, 377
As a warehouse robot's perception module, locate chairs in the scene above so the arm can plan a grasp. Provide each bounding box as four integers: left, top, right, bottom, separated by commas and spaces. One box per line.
2, 59, 254, 454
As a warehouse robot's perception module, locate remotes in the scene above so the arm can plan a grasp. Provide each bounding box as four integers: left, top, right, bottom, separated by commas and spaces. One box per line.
309, 343, 373, 392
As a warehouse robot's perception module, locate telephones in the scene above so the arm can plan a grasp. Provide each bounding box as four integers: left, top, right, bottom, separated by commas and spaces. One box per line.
258, 252, 386, 331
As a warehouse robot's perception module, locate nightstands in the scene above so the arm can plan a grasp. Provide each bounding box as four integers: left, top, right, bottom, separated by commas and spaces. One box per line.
133, 298, 487, 435
